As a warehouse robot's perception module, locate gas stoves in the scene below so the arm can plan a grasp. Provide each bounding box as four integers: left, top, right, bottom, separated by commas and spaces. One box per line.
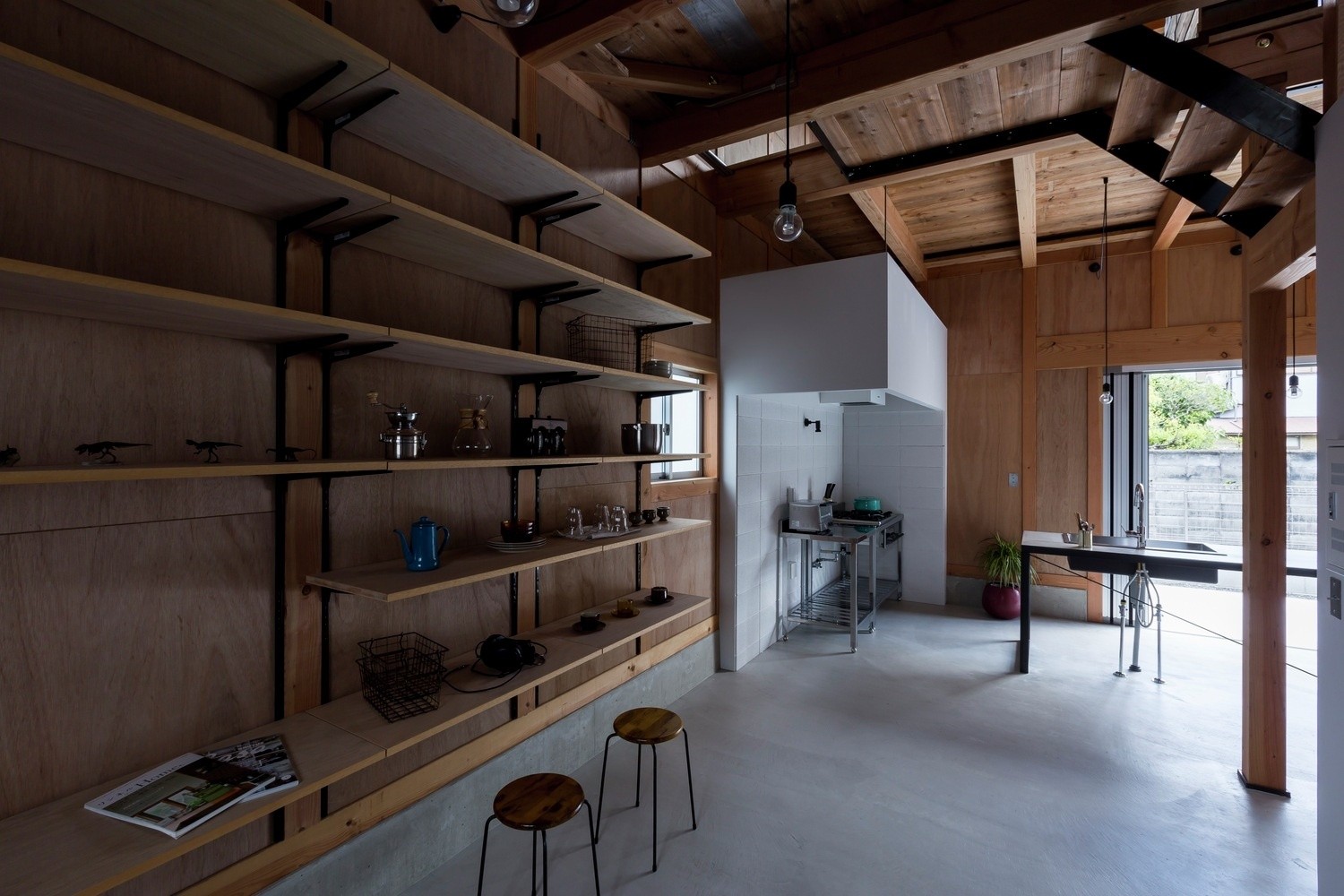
827, 506, 895, 533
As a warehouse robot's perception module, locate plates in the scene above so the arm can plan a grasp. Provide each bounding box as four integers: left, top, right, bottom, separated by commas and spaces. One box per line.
486, 535, 547, 553
643, 358, 672, 379
645, 594, 674, 605
572, 621, 608, 633
610, 606, 640, 618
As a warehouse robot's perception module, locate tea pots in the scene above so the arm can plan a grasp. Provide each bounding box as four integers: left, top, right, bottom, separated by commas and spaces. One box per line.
394, 513, 451, 571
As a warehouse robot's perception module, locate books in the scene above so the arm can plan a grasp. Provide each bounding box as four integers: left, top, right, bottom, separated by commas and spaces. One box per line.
84, 733, 300, 839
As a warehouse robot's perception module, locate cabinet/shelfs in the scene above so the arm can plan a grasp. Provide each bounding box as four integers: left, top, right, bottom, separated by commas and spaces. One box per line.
0, 0, 717, 896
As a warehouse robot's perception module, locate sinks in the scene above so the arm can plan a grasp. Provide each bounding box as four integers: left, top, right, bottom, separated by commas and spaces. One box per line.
1062, 532, 1228, 585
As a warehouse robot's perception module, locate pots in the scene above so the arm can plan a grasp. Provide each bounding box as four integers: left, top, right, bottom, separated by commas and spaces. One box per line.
853, 495, 881, 511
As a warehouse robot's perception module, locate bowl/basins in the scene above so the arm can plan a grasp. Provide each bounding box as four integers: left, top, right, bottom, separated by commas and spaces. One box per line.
500, 519, 533, 543
620, 421, 664, 454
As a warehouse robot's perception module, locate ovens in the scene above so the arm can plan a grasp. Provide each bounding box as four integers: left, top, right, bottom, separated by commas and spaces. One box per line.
789, 497, 833, 532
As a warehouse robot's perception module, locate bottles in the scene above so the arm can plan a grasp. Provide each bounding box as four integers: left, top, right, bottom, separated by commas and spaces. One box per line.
450, 406, 497, 458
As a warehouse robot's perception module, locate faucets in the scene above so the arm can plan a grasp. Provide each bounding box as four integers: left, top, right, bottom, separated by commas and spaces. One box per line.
1133, 482, 1148, 549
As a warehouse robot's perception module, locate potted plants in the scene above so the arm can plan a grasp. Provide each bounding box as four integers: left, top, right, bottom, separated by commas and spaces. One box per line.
971, 530, 1045, 621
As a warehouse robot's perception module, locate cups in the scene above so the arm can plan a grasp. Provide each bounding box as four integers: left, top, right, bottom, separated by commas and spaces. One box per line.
656, 506, 671, 522
617, 598, 634, 615
630, 511, 643, 527
594, 503, 610, 533
651, 586, 668, 600
580, 611, 601, 627
1078, 527, 1093, 549
609, 506, 629, 534
642, 508, 656, 524
565, 505, 584, 537
